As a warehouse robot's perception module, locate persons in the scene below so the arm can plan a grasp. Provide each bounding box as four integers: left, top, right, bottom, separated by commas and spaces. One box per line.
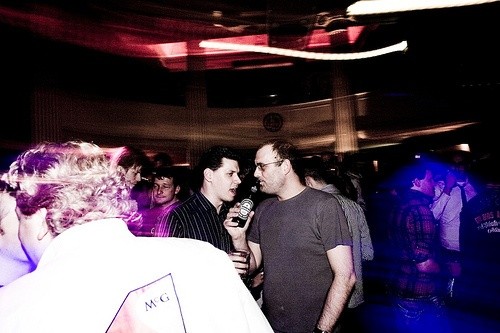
115, 138, 500, 333
0, 140, 274, 333
0, 170, 37, 288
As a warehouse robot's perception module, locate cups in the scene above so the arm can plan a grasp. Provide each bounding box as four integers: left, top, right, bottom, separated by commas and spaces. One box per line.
230, 248, 251, 281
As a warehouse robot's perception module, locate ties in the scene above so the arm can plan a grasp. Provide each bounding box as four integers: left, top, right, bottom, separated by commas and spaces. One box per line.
456, 183, 469, 206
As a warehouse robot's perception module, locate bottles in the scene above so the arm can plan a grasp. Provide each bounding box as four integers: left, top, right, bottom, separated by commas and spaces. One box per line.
231, 179, 260, 228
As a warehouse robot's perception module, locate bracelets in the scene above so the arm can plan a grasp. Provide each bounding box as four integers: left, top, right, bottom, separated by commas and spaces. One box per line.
313, 328, 328, 333
444, 187, 453, 191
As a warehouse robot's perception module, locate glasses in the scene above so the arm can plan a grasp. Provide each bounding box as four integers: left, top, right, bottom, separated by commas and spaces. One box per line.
256, 160, 283, 170
